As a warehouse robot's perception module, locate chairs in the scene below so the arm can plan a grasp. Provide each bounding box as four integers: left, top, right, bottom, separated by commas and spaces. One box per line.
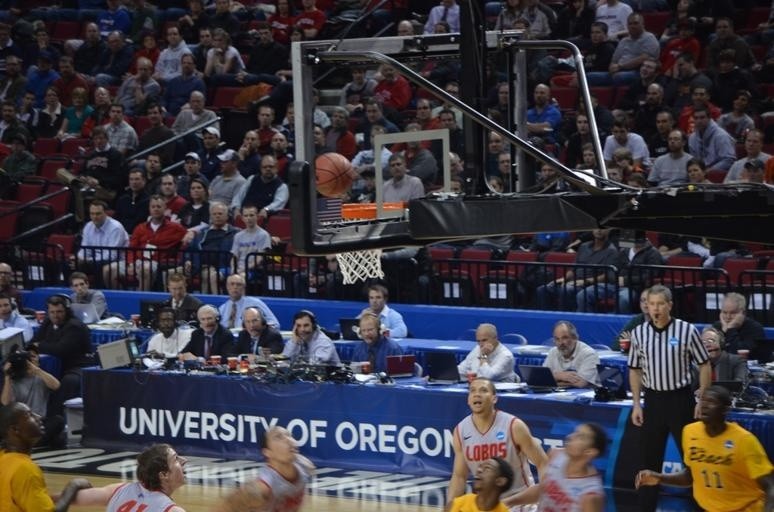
1, 5, 772, 318
539, 335, 554, 345
500, 332, 528, 345
460, 327, 476, 340
590, 343, 612, 350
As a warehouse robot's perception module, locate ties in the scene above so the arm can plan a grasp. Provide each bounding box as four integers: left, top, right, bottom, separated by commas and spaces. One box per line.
250, 339, 255, 354
303, 342, 308, 352
174, 300, 179, 309
205, 336, 211, 360
228, 303, 235, 327
367, 350, 375, 374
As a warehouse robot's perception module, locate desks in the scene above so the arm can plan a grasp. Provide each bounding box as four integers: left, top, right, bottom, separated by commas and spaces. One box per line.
84, 353, 773, 480
86, 319, 629, 377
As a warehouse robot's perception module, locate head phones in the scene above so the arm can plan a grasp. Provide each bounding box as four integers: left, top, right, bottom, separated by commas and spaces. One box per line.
43, 292, 72, 314
0, 294, 16, 310
355, 312, 386, 339
718, 330, 732, 349
293, 310, 317, 331
194, 302, 221, 323
240, 306, 267, 328
149, 307, 180, 330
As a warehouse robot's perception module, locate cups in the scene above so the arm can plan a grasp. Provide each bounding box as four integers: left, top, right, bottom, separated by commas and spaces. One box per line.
619, 339, 631, 356
131, 314, 141, 326
360, 362, 371, 375
36, 311, 46, 323
738, 350, 750, 359
241, 361, 249, 375
210, 354, 222, 366
227, 357, 239, 372
467, 372, 476, 385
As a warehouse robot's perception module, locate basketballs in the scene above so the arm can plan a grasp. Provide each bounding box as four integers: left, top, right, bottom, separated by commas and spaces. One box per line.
315, 153, 353, 198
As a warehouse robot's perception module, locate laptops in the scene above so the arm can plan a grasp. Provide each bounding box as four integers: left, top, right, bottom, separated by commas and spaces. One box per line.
381, 354, 644, 397
139, 301, 160, 324
340, 318, 363, 340
69, 303, 101, 325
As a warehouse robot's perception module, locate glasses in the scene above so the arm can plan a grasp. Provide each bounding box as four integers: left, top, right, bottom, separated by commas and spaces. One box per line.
702, 339, 714, 345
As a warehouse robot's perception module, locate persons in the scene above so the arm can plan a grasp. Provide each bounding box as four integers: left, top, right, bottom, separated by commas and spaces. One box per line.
0, 0, 774, 511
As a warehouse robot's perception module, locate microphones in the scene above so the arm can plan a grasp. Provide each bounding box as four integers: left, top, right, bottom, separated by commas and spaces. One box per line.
205, 322, 217, 329
252, 327, 268, 334
5, 308, 13, 314
302, 329, 315, 335
55, 314, 68, 328
708, 348, 721, 352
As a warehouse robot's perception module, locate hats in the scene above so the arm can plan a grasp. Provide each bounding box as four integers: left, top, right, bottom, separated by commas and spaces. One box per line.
360, 167, 375, 179
215, 149, 240, 163
185, 152, 200, 161
204, 127, 220, 139
